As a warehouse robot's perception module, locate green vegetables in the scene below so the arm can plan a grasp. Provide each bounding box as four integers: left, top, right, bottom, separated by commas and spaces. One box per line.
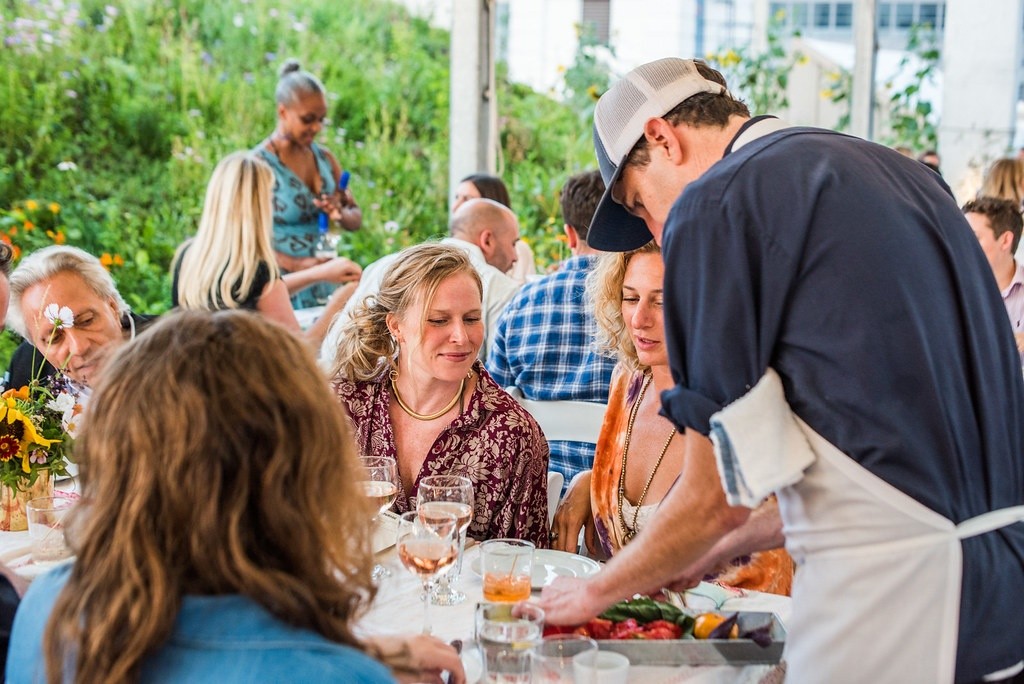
600, 597, 696, 635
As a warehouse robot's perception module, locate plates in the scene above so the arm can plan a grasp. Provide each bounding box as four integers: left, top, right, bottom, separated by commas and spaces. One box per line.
540, 611, 788, 666
470, 549, 601, 591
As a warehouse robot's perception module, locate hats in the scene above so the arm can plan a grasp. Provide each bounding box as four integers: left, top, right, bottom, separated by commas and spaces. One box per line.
585, 58, 728, 252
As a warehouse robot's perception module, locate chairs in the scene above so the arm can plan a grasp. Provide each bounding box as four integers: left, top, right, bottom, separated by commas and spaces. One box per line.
503, 386, 609, 555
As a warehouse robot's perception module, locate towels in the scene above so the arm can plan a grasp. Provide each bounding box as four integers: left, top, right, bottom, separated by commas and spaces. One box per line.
709, 367, 815, 509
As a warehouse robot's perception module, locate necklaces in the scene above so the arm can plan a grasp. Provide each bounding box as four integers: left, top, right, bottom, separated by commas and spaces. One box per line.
389, 371, 464, 421
618, 371, 678, 546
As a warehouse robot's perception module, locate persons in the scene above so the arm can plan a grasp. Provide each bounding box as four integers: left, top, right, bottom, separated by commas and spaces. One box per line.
510, 57, 1024, 684
328, 242, 551, 550
320, 198, 520, 363
976, 158, 1023, 221
921, 150, 941, 168
484, 167, 621, 504
251, 60, 363, 310
170, 152, 363, 361
550, 238, 795, 597
450, 173, 537, 284
8, 246, 165, 397
958, 194, 1023, 364
5, 310, 467, 684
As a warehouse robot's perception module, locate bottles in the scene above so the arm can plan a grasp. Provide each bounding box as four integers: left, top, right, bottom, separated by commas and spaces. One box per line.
331, 172, 352, 210
314, 211, 342, 305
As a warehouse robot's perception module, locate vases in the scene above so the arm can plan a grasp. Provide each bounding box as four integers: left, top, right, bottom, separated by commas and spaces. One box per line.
28, 468, 54, 524
0, 472, 28, 532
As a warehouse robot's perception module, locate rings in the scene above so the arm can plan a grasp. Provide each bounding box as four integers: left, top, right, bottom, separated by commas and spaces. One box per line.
549, 531, 558, 539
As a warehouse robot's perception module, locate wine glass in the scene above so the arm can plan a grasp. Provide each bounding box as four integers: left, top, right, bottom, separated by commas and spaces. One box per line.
350, 455, 400, 582
416, 474, 475, 605
396, 510, 458, 637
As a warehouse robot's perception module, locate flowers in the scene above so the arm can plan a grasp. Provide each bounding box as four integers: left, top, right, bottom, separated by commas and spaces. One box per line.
0, 282, 92, 500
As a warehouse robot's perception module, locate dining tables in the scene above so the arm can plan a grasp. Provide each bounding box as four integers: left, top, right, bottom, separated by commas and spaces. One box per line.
350, 516, 791, 684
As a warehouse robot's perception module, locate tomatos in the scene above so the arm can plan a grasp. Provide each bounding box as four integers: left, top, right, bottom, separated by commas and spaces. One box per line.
540, 617, 684, 640
693, 613, 739, 639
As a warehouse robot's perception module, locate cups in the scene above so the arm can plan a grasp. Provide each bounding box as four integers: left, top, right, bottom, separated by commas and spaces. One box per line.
573, 649, 630, 684
413, 516, 467, 581
526, 273, 545, 282
528, 633, 600, 684
479, 537, 535, 605
475, 602, 546, 684
24, 496, 74, 567
